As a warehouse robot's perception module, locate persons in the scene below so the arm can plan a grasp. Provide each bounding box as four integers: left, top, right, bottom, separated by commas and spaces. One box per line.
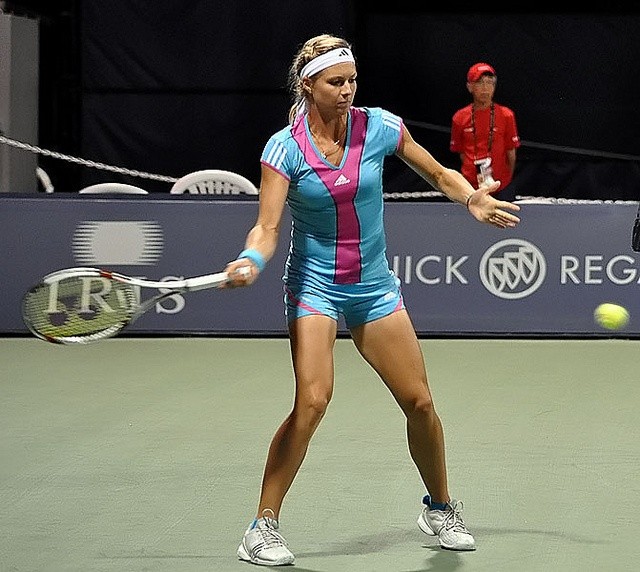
451, 62, 519, 202
219, 35, 521, 566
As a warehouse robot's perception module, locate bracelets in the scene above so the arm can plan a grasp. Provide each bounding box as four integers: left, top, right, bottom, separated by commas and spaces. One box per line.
237, 249, 265, 273
466, 190, 477, 211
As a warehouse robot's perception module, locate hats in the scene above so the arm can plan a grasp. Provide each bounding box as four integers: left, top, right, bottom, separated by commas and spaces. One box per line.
467, 62, 495, 82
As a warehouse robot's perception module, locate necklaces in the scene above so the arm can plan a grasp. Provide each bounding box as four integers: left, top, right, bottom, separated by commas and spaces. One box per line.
307, 119, 348, 160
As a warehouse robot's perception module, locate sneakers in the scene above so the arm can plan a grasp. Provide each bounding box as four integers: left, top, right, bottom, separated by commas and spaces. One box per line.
237, 508, 295, 566
417, 495, 476, 550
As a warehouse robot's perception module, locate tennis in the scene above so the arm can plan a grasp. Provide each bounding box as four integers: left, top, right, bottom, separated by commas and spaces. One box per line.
594, 302, 631, 332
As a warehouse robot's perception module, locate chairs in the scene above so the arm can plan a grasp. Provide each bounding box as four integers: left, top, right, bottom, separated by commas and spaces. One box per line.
169, 168, 259, 199
